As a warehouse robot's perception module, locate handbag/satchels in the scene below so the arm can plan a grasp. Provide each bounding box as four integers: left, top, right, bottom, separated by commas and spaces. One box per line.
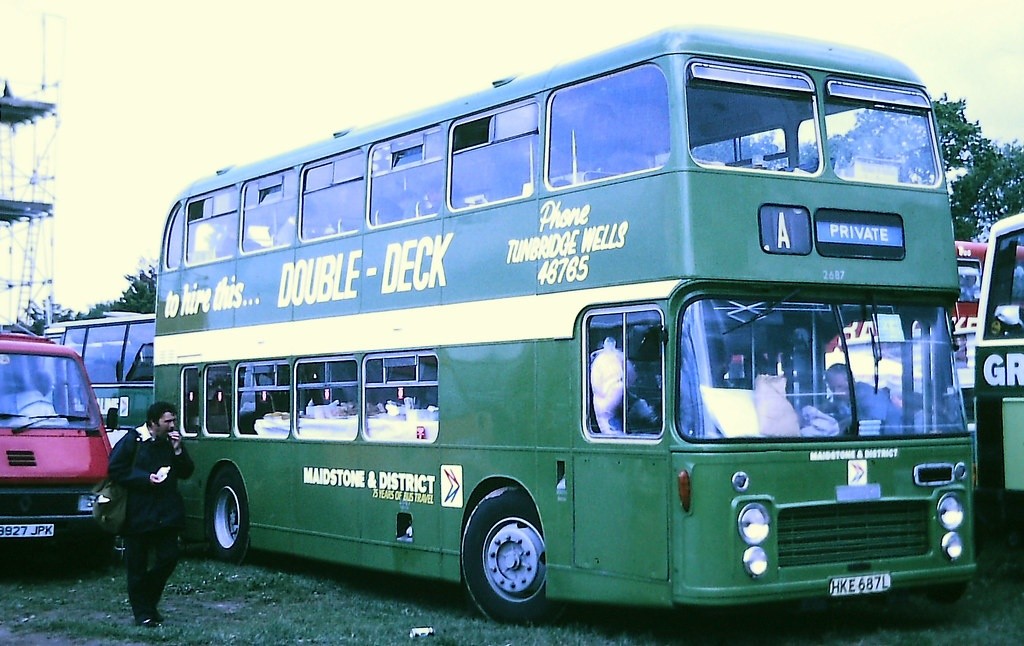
91, 477, 127, 532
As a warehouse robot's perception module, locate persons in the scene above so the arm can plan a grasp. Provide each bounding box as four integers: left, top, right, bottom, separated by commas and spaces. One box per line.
819, 363, 901, 436
107, 402, 196, 630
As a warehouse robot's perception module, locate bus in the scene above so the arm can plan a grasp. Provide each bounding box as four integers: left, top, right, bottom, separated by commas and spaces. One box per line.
0, 321, 121, 569
163, 26, 976, 631
42, 312, 158, 455
824, 241, 1024, 407
973, 210, 1024, 564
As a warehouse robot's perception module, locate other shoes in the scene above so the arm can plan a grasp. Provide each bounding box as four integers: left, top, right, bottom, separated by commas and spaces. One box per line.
142, 619, 157, 626
156, 614, 164, 622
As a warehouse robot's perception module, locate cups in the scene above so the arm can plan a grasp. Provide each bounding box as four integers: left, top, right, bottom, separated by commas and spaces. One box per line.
261, 397, 437, 423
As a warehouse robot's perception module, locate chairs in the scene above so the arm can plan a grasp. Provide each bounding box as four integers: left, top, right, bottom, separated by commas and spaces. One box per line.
1, 384, 61, 424
268, 195, 439, 248
